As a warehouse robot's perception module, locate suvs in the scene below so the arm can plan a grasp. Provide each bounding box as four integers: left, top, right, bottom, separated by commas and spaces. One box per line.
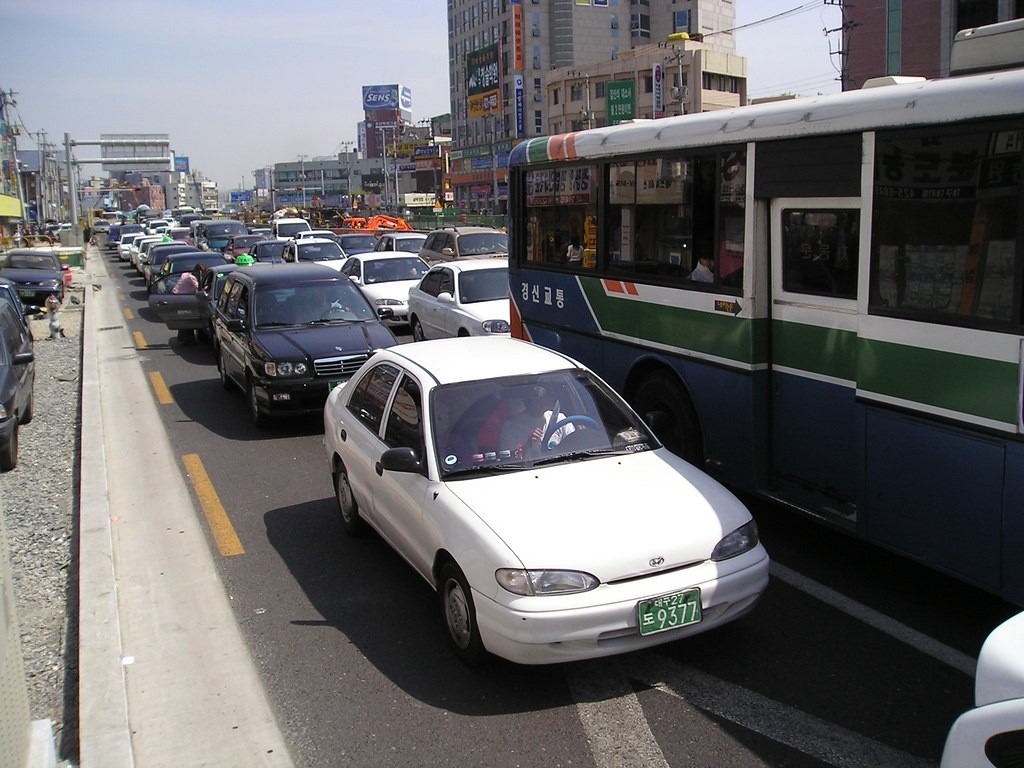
211, 261, 400, 426
418, 225, 509, 268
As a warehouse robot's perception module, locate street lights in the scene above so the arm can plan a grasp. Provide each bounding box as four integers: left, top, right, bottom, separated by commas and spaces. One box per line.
668, 32, 690, 115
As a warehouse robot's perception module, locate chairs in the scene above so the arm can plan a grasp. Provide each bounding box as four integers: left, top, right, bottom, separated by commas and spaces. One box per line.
257, 293, 288, 324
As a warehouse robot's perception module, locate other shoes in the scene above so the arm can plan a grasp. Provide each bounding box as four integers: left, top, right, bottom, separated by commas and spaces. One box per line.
184, 341, 200, 346
178, 337, 184, 342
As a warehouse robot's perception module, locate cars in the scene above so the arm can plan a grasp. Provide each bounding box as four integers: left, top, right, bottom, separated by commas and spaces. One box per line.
321, 334, 770, 666
338, 251, 432, 327
90, 201, 433, 356
0, 279, 36, 472
939, 607, 1024, 767
408, 258, 511, 342
0, 248, 68, 305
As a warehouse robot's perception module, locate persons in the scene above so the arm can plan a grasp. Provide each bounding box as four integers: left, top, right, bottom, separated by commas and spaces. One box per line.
237, 241, 245, 248
564, 233, 585, 267
302, 290, 353, 322
12, 224, 42, 248
691, 244, 716, 284
171, 263, 209, 345
499, 384, 587, 461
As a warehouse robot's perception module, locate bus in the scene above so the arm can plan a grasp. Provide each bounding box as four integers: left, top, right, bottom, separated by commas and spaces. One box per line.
509, 16, 1024, 607
